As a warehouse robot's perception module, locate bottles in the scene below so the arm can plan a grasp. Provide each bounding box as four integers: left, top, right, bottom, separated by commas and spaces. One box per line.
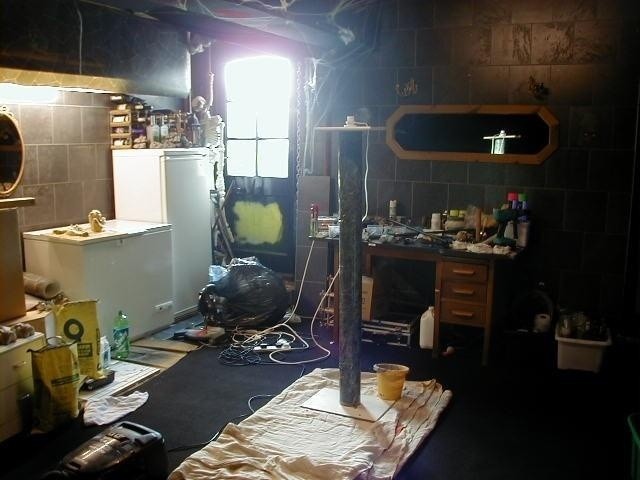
111, 310, 130, 360
492, 191, 529, 245
110, 102, 184, 147
389, 200, 398, 222
431, 212, 441, 230
557, 306, 608, 343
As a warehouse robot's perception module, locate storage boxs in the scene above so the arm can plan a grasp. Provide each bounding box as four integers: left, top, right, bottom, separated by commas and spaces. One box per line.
555, 324, 612, 373
0, 208, 26, 323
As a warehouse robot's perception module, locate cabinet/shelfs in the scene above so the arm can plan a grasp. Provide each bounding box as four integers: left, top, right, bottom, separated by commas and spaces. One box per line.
0, 333, 47, 442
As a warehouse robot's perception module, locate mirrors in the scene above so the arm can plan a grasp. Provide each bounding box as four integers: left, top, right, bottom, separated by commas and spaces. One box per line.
385, 105, 561, 164
0, 107, 26, 198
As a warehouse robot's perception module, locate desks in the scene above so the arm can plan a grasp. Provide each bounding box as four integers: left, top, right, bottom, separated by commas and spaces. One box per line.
308, 235, 516, 364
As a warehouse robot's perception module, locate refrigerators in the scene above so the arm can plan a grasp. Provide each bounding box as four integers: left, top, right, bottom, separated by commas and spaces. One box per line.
111, 146, 213, 325
21, 218, 177, 354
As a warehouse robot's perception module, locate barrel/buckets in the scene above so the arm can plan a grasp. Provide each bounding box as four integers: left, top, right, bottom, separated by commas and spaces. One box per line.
419, 306, 434, 349
374, 364, 409, 399
